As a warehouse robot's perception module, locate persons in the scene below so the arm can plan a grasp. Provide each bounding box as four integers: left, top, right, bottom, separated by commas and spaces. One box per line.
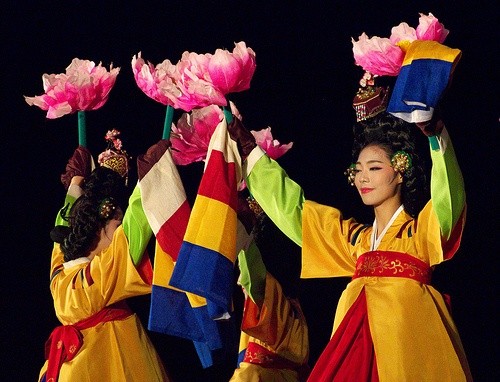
38, 108, 309, 382
227, 109, 474, 382
